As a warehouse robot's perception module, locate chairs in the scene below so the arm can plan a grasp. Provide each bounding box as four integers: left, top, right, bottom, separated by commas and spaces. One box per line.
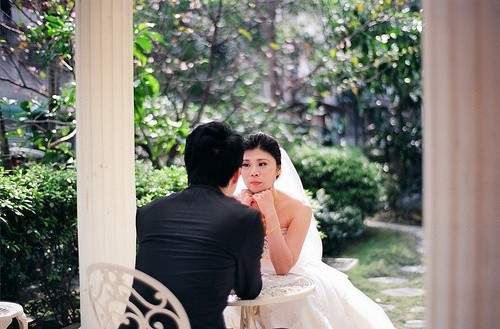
86, 264, 191, 329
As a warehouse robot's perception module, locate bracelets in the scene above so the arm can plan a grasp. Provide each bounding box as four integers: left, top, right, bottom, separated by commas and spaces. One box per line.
265, 223, 280, 234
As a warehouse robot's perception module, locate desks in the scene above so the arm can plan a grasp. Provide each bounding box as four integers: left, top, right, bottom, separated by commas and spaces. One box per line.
222, 269, 315, 329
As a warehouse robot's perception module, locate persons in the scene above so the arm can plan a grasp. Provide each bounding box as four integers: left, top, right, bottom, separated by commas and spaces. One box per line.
229, 132, 395, 329
133, 121, 269, 329
